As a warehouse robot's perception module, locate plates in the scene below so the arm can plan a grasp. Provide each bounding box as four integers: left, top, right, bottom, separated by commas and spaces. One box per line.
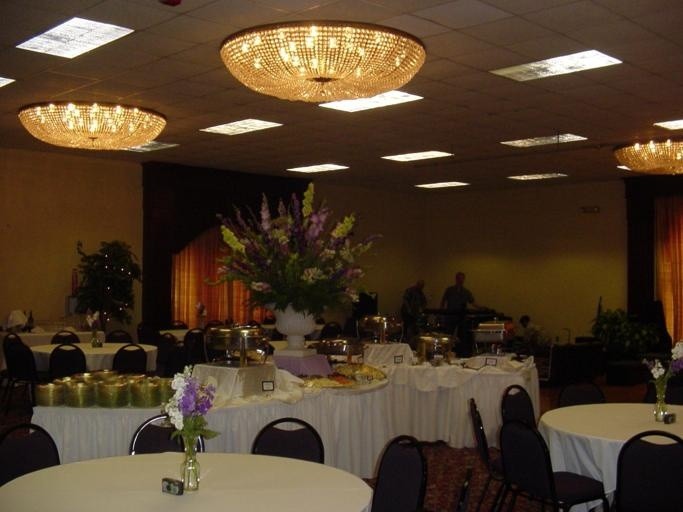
33, 370, 173, 409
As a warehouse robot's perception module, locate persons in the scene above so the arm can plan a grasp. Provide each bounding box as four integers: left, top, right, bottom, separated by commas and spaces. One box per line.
400, 279, 428, 345
517, 315, 547, 347
439, 272, 483, 339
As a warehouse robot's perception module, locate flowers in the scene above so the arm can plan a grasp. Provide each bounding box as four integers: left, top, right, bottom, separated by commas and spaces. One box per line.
201, 177, 383, 323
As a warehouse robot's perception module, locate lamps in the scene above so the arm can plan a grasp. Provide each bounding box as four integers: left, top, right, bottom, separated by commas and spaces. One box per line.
612, 135, 683, 175
218, 18, 427, 104
17, 100, 167, 151
